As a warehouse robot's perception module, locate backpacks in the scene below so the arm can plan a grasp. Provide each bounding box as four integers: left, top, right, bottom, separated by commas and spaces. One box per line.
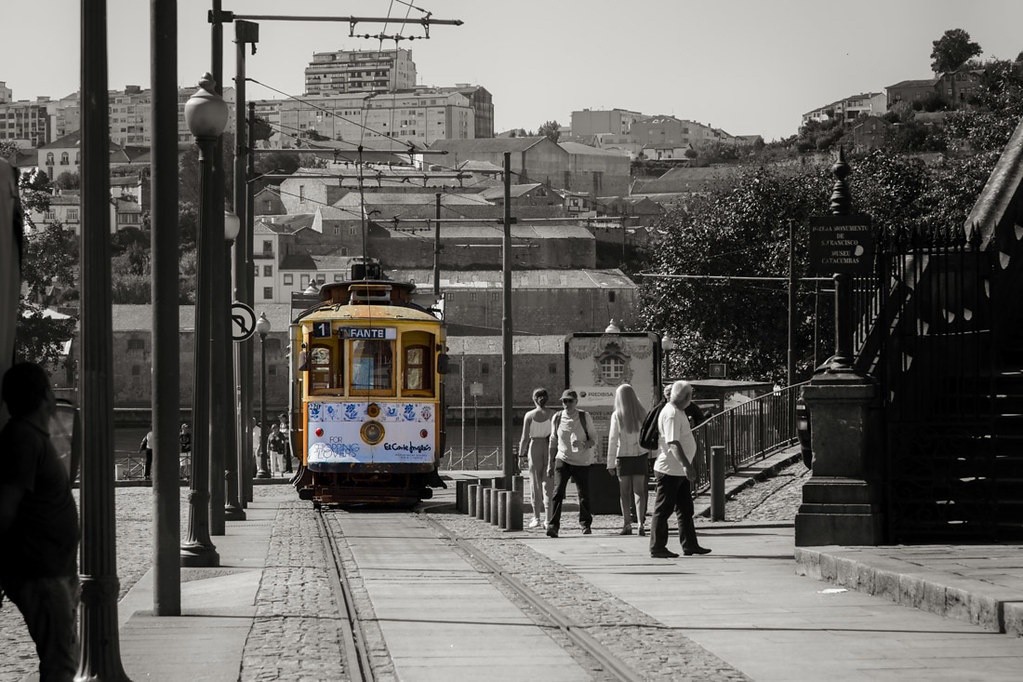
639, 403, 665, 450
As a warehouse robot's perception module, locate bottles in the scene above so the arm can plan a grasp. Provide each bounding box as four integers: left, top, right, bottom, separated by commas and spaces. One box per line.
570, 432, 578, 452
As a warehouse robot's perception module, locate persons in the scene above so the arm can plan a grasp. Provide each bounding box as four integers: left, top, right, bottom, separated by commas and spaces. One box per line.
517, 388, 560, 530
0, 363, 83, 682
664, 384, 705, 496
606, 384, 649, 536
142, 413, 292, 482
547, 390, 598, 538
649, 380, 711, 559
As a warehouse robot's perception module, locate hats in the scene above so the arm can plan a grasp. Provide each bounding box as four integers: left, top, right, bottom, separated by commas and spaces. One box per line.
559, 389, 577, 400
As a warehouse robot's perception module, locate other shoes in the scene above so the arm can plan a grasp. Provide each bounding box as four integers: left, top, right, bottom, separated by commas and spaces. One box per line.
581, 525, 591, 534
684, 546, 712, 555
543, 521, 547, 529
529, 518, 540, 527
546, 528, 558, 538
651, 547, 680, 558
620, 529, 632, 534
638, 529, 645, 536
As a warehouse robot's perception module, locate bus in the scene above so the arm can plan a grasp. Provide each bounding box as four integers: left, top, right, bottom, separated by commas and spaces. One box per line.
284, 253, 450, 519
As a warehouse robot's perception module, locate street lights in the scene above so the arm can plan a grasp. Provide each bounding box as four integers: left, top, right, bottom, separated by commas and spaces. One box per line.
180, 71, 230, 568
254, 311, 272, 478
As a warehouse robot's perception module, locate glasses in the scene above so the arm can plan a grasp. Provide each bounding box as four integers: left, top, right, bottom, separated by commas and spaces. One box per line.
666, 393, 670, 396
563, 399, 573, 403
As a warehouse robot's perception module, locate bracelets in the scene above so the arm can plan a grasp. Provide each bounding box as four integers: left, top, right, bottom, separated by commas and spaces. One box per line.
581, 441, 586, 447
518, 456, 523, 458
